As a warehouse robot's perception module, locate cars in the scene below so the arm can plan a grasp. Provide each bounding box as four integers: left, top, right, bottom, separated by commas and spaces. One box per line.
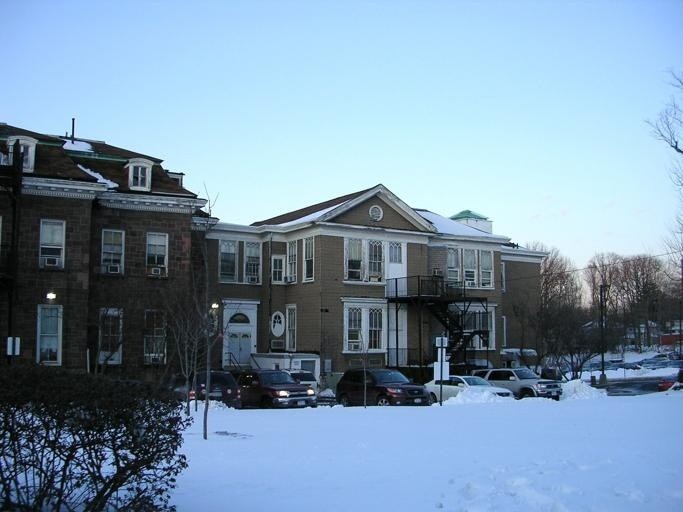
171, 349, 322, 405
336, 362, 563, 405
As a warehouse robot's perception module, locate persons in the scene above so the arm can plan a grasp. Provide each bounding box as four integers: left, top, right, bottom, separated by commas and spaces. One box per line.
674, 367, 683, 390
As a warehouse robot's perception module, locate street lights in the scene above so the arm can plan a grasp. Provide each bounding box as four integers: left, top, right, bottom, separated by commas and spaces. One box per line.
595, 283, 611, 375
44, 291, 56, 368
